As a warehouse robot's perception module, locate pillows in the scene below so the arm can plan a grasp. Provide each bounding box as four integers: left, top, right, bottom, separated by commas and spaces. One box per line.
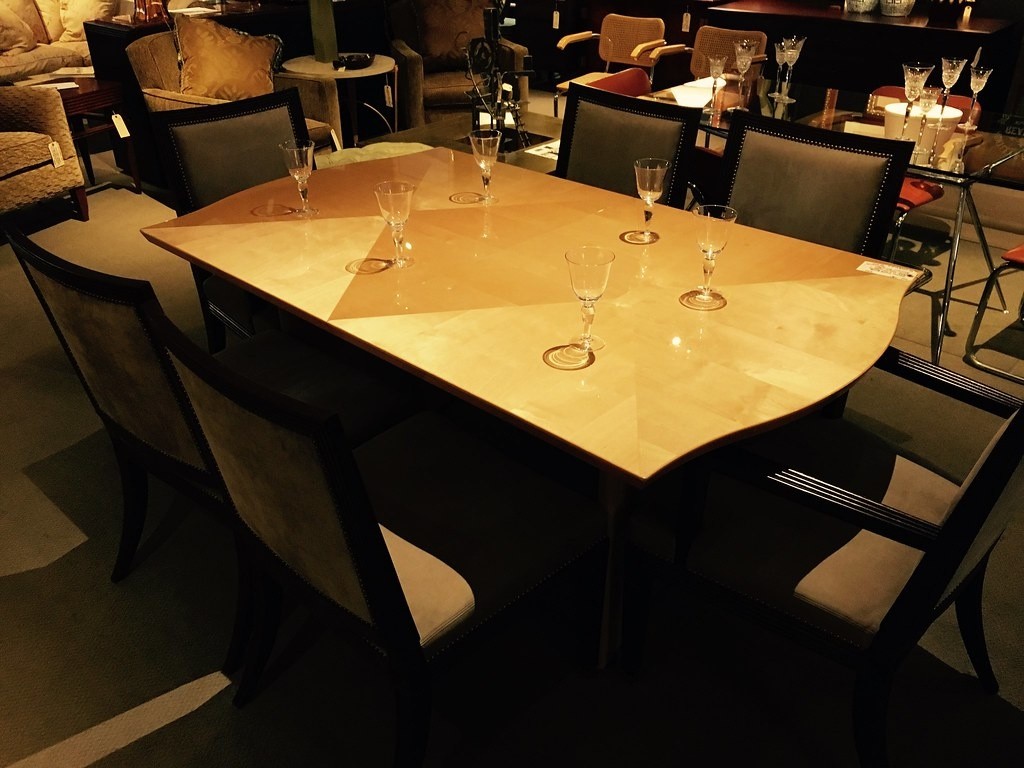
410, 0, 489, 71
0, 2, 37, 56
172, 11, 281, 102
59, 0, 117, 41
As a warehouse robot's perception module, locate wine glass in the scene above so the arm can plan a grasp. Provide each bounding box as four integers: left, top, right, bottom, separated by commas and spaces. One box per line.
700, 34, 808, 127
565, 247, 616, 351
372, 180, 416, 270
678, 204, 739, 312
894, 56, 993, 174
628, 157, 671, 243
278, 139, 319, 218
468, 129, 503, 206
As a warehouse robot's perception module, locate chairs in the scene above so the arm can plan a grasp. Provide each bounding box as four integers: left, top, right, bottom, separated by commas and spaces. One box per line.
2, 223, 1024, 768
554, 13, 1024, 387
147, 86, 316, 355
0, 85, 90, 222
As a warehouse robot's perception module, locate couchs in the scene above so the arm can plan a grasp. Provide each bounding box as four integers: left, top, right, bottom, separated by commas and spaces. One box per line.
381, 0, 531, 128
0, 0, 210, 83
121, 28, 342, 206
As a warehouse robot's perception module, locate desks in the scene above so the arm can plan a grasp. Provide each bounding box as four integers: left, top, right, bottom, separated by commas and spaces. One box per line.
138, 145, 924, 673
25, 77, 142, 196
83, 3, 310, 189
280, 52, 399, 145
635, 73, 1024, 366
709, 0, 1024, 133
